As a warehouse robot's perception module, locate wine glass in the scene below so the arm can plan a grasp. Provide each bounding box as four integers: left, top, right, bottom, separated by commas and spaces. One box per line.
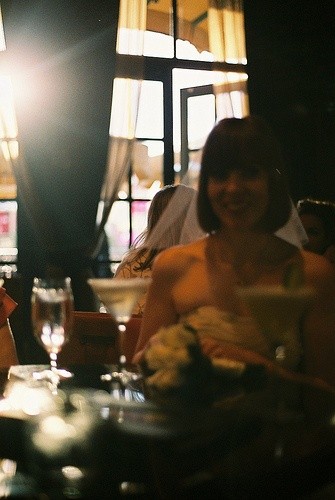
29, 275, 73, 377
88, 278, 151, 383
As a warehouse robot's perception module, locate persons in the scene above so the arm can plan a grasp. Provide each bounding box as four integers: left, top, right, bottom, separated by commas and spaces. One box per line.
298, 200, 335, 264
112, 182, 198, 317
131, 115, 335, 396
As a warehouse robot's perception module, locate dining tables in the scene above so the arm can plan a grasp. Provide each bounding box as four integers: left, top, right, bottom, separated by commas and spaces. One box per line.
1, 344, 335, 500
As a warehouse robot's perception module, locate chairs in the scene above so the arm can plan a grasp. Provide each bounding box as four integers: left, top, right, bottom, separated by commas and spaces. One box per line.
70, 307, 143, 366
